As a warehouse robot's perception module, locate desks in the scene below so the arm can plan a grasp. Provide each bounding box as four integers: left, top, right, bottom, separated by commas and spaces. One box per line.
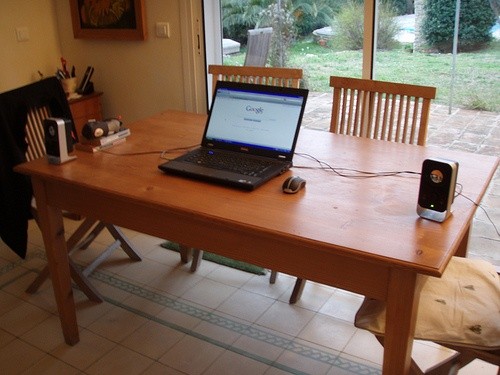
13, 109, 500, 375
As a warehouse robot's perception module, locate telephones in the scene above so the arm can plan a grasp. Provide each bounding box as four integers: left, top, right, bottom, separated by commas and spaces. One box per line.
75, 65, 94, 95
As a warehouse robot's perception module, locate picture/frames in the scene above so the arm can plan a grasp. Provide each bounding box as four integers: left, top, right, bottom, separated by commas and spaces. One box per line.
69, 0, 149, 42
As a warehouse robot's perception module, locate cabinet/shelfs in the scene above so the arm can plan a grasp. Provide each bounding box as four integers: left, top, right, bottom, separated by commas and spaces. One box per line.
68, 91, 105, 137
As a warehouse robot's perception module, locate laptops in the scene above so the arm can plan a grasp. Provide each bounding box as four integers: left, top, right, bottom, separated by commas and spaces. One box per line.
157, 80, 309, 193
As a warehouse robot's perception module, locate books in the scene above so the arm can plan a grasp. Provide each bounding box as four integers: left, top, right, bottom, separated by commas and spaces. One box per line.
75, 128, 131, 153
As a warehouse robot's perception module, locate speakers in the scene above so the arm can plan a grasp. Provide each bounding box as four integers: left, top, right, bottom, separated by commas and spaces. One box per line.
43, 117, 78, 165
416, 157, 459, 222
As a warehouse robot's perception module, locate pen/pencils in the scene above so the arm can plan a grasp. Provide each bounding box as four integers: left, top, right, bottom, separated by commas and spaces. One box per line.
55, 57, 76, 80
37, 70, 45, 80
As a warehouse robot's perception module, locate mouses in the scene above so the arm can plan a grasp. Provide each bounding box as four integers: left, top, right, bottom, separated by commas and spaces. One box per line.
282, 175, 306, 194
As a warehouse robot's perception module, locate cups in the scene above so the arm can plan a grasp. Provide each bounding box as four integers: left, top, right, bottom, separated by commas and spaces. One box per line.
62, 78, 77, 93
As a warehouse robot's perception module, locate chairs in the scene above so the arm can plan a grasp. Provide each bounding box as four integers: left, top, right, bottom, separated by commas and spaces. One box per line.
190, 65, 500, 375
0, 76, 143, 304
